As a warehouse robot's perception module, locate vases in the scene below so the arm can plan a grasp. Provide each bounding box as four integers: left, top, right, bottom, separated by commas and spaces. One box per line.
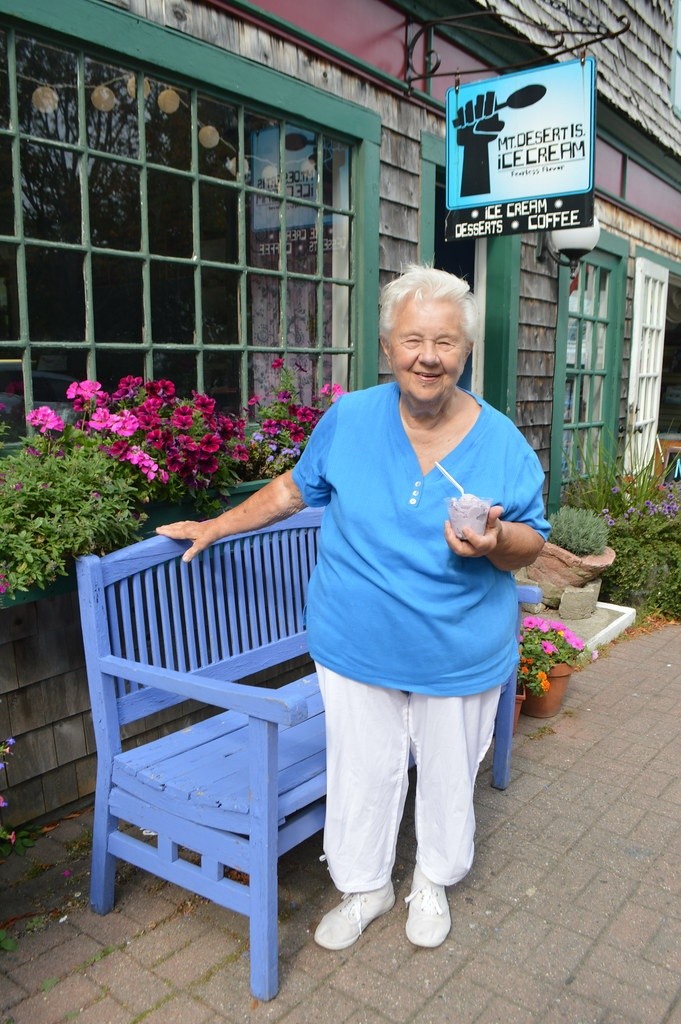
520, 660, 575, 719
512, 684, 525, 737
134, 488, 214, 535
215, 458, 275, 507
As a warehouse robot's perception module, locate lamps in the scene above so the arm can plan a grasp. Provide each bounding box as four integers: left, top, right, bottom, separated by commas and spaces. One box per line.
32, 86, 60, 113
157, 90, 181, 113
227, 155, 251, 177
126, 77, 151, 98
534, 217, 601, 280
90, 86, 115, 112
261, 164, 279, 187
199, 125, 220, 149
301, 160, 315, 178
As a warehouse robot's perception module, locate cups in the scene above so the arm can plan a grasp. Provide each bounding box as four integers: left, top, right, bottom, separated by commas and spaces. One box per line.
445, 495, 492, 539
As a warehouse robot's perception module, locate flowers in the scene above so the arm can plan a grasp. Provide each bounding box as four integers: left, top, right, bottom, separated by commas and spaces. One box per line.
517, 648, 551, 699
33, 377, 229, 509
518, 616, 599, 671
232, 357, 352, 482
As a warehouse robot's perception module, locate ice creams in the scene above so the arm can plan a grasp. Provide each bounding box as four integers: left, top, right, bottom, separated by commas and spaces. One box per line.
443, 493, 492, 540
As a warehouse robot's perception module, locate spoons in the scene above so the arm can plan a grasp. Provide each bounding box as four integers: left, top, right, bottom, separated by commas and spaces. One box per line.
435, 461, 479, 501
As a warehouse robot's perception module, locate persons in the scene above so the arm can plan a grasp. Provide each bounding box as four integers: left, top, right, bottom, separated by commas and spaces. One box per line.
151, 262, 553, 949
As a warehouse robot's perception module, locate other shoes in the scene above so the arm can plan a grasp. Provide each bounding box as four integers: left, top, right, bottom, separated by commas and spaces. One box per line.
404, 862, 451, 948
313, 880, 395, 950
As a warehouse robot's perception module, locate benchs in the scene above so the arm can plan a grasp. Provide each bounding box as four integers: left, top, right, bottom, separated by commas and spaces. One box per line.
76, 507, 518, 998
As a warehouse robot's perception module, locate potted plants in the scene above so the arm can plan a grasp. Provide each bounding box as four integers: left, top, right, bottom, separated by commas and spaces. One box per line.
527, 505, 616, 609
